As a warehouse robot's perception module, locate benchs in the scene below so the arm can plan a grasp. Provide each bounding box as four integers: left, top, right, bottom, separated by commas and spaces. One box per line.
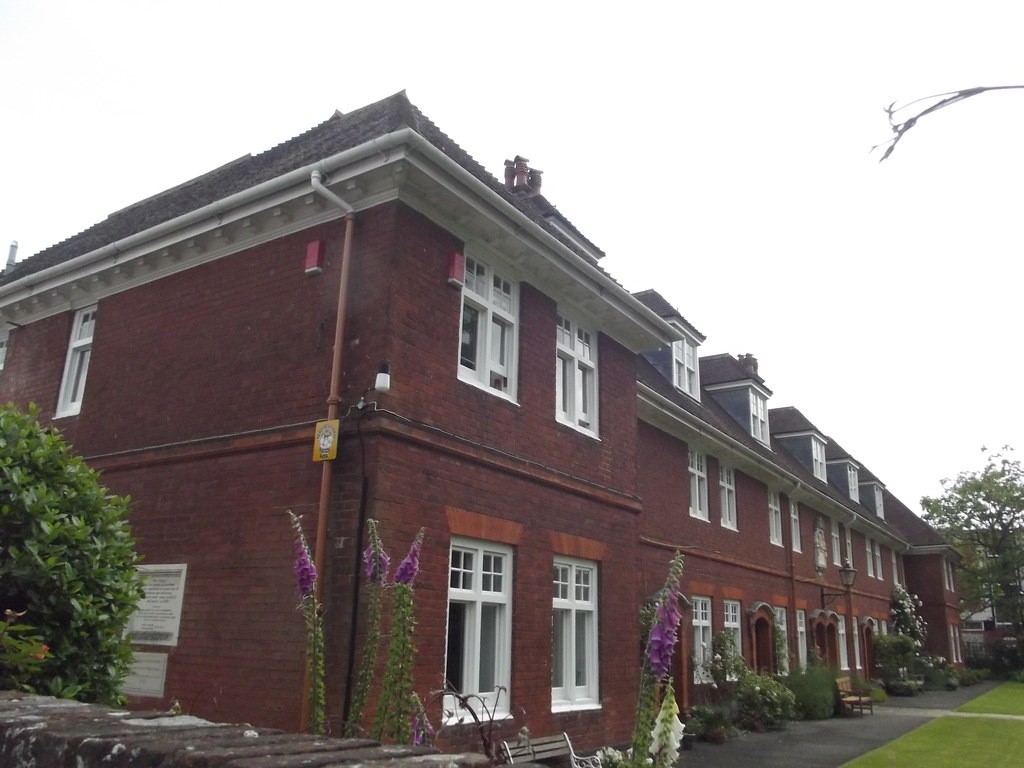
898, 667, 925, 693
500, 732, 602, 768
835, 676, 873, 719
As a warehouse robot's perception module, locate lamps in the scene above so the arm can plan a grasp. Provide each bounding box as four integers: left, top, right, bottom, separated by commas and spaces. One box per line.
358, 362, 391, 410
820, 561, 859, 612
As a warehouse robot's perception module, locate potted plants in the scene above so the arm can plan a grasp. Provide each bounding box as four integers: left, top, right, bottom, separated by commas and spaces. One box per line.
705, 710, 726, 744
683, 717, 701, 751
